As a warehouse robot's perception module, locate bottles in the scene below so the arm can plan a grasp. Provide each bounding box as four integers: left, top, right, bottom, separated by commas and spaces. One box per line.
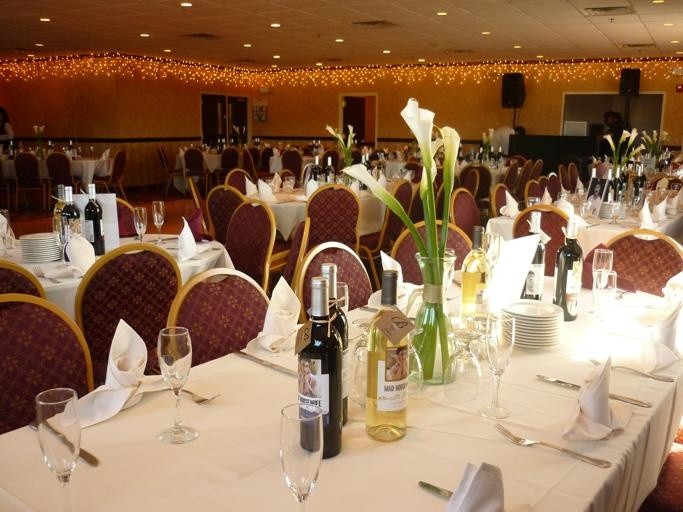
69, 139, 75, 161
614, 168, 622, 200
320, 262, 350, 426
478, 147, 484, 165
324, 156, 335, 185
52, 183, 65, 233
552, 217, 583, 324
520, 210, 546, 299
317, 139, 322, 154
47, 138, 53, 155
84, 183, 106, 256
607, 169, 616, 201
488, 147, 497, 168
633, 164, 646, 195
591, 168, 597, 179
497, 146, 504, 167
61, 185, 81, 263
312, 139, 317, 156
217, 138, 226, 151
8, 139, 15, 160
297, 277, 343, 460
231, 139, 234, 147
312, 154, 322, 183
458, 143, 463, 161
365, 271, 409, 442
255, 137, 265, 150
655, 146, 672, 173
377, 152, 386, 183
359, 153, 371, 190
459, 225, 492, 320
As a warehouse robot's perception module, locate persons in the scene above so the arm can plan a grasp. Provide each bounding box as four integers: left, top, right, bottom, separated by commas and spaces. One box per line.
70, 219, 82, 234
598, 111, 633, 158
386, 348, 409, 380
527, 272, 539, 294
298, 359, 319, 397
354, 145, 369, 164
588, 184, 601, 216
0, 106, 15, 153
366, 153, 380, 170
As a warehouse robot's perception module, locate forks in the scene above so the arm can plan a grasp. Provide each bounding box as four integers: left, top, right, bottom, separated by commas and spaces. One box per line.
150, 364, 221, 407
32, 265, 62, 287
490, 421, 612, 471
587, 357, 680, 386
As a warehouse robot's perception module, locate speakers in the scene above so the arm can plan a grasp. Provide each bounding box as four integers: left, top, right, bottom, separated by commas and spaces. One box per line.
620, 69, 640, 97
502, 73, 525, 108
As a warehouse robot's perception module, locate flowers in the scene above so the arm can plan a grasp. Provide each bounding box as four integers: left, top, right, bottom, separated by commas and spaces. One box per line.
337, 88, 463, 383
481, 127, 495, 163
639, 125, 669, 160
321, 121, 359, 163
599, 125, 648, 167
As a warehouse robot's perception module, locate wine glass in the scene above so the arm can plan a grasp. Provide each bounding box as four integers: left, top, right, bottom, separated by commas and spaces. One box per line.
133, 207, 147, 244
151, 201, 165, 246
671, 184, 682, 191
480, 312, 516, 422
435, 243, 454, 291
276, 402, 324, 510
484, 231, 500, 278
34, 388, 81, 512
575, 187, 586, 207
353, 318, 369, 409
591, 249, 615, 317
556, 191, 572, 204
284, 175, 296, 189
407, 325, 425, 402
52, 216, 70, 269
627, 195, 643, 223
283, 182, 293, 203
265, 179, 277, 193
609, 200, 621, 227
443, 330, 484, 406
579, 200, 593, 227
593, 270, 618, 337
155, 325, 199, 445
466, 314, 490, 411
527, 197, 540, 208
337, 280, 349, 317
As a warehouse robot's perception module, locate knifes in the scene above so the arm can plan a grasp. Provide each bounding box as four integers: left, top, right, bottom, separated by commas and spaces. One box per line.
35, 414, 100, 467
535, 372, 653, 416
229, 347, 296, 378
145, 237, 178, 242
419, 481, 451, 500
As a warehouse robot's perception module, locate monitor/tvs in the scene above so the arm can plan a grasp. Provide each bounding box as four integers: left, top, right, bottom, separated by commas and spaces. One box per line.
564, 121, 587, 136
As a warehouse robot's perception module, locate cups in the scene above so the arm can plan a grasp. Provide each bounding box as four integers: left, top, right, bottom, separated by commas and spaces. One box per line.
391, 172, 401, 186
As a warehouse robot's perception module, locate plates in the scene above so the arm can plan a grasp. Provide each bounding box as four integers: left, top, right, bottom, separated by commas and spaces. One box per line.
19, 232, 62, 264
502, 297, 565, 352
